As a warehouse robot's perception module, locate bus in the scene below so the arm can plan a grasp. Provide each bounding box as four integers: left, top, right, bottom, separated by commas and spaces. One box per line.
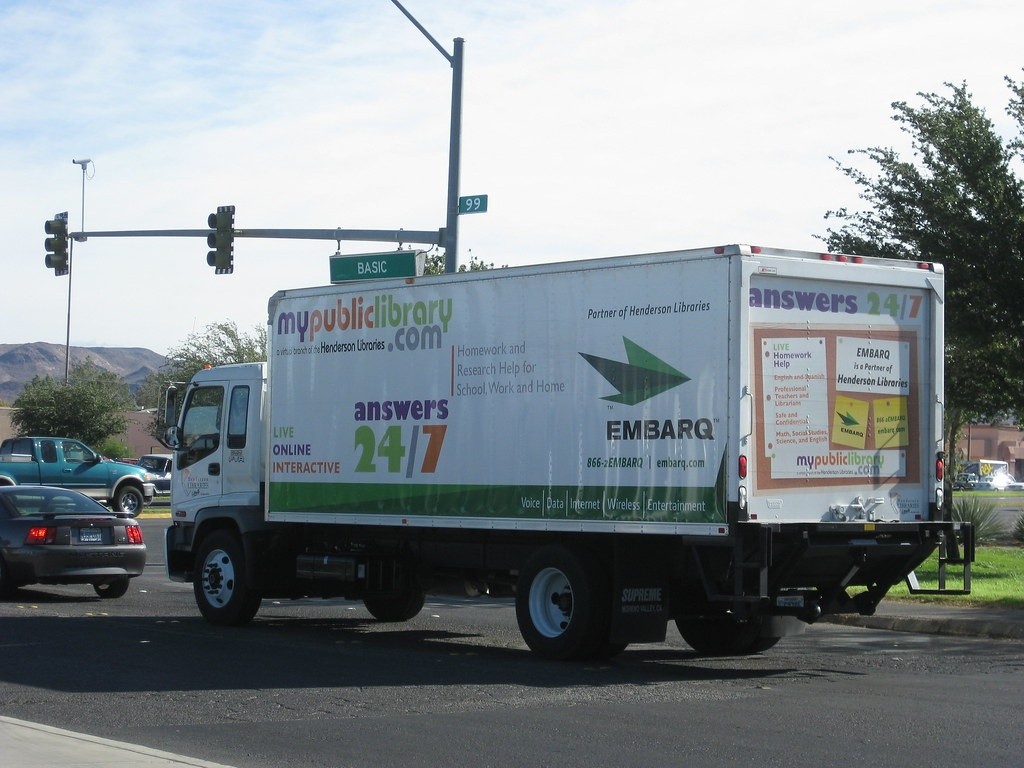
963, 460, 1009, 476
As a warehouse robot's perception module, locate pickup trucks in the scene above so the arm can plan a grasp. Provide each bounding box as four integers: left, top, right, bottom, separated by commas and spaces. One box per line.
1, 436, 155, 519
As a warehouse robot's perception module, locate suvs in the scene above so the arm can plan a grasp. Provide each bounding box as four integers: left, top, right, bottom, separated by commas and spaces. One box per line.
953, 473, 976, 491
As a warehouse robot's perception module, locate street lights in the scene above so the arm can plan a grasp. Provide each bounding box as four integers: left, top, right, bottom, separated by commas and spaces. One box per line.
45, 211, 70, 278
206, 204, 236, 274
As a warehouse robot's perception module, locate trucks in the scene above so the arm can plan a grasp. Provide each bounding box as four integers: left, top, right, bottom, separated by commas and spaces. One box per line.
154, 244, 977, 665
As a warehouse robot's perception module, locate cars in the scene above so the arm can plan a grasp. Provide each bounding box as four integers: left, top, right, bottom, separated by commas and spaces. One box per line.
1005, 478, 1024, 491
138, 453, 173, 504
0, 485, 148, 599
972, 473, 1015, 491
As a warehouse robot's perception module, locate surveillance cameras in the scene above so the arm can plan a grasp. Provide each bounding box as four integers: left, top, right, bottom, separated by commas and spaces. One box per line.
72, 156, 92, 164
